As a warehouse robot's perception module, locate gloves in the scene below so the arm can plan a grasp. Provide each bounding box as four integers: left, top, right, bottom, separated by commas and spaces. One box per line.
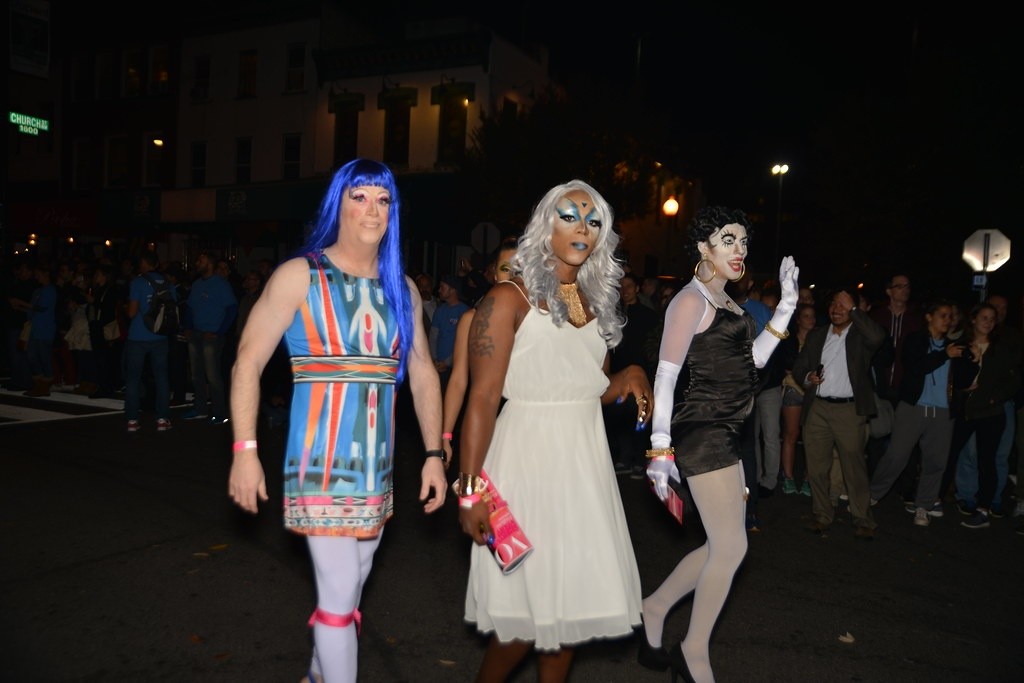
645, 358, 682, 503
753, 256, 799, 369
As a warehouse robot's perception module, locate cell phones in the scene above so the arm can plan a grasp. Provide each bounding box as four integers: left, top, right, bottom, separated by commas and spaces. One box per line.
954, 341, 970, 354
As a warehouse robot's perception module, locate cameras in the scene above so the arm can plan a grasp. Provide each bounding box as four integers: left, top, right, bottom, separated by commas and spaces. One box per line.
817, 364, 826, 379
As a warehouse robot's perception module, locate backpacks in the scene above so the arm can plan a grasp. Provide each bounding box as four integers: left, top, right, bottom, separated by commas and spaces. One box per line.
139, 272, 181, 335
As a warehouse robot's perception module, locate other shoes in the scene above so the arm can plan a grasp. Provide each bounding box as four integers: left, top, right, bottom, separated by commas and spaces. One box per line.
927, 499, 943, 517
800, 480, 812, 498
7, 378, 229, 431
960, 510, 990, 528
783, 479, 799, 495
954, 496, 974, 516
913, 506, 931, 526
805, 517, 827, 535
614, 460, 642, 479
854, 524, 873, 542
846, 495, 877, 515
905, 497, 916, 514
989, 500, 1016, 517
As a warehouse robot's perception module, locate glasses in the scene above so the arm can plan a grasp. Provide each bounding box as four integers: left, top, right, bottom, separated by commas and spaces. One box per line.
890, 281, 914, 289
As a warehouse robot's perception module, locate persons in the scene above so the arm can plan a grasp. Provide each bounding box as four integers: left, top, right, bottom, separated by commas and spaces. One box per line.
226, 156, 450, 683
636, 206, 801, 682
722, 270, 1023, 539
602, 261, 691, 481
0, 253, 139, 397
121, 248, 295, 434
458, 179, 654, 683
411, 236, 520, 469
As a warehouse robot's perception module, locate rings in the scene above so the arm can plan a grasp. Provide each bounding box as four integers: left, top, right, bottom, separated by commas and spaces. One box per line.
636, 395, 646, 405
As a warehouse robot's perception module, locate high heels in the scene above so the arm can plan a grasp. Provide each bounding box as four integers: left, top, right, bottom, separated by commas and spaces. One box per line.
670, 643, 697, 682
633, 610, 672, 673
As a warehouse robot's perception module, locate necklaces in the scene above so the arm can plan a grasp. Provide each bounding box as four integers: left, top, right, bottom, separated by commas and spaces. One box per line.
556, 278, 589, 324
712, 287, 734, 310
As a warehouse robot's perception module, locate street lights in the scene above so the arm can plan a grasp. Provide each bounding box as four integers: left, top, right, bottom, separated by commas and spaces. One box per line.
770, 163, 789, 264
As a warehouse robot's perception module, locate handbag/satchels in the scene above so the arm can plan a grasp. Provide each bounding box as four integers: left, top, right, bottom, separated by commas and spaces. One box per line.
102, 321, 120, 340
20, 321, 32, 343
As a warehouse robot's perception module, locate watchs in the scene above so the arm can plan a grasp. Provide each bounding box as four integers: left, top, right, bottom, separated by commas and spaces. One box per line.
426, 448, 447, 462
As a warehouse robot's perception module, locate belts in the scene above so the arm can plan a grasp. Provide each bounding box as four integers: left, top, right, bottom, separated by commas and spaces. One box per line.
815, 394, 857, 403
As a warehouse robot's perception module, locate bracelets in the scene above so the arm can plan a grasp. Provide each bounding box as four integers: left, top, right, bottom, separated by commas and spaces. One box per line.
443, 433, 453, 441
459, 494, 481, 510
645, 446, 676, 457
452, 471, 484, 495
765, 321, 791, 339
652, 455, 675, 461
233, 439, 257, 452
